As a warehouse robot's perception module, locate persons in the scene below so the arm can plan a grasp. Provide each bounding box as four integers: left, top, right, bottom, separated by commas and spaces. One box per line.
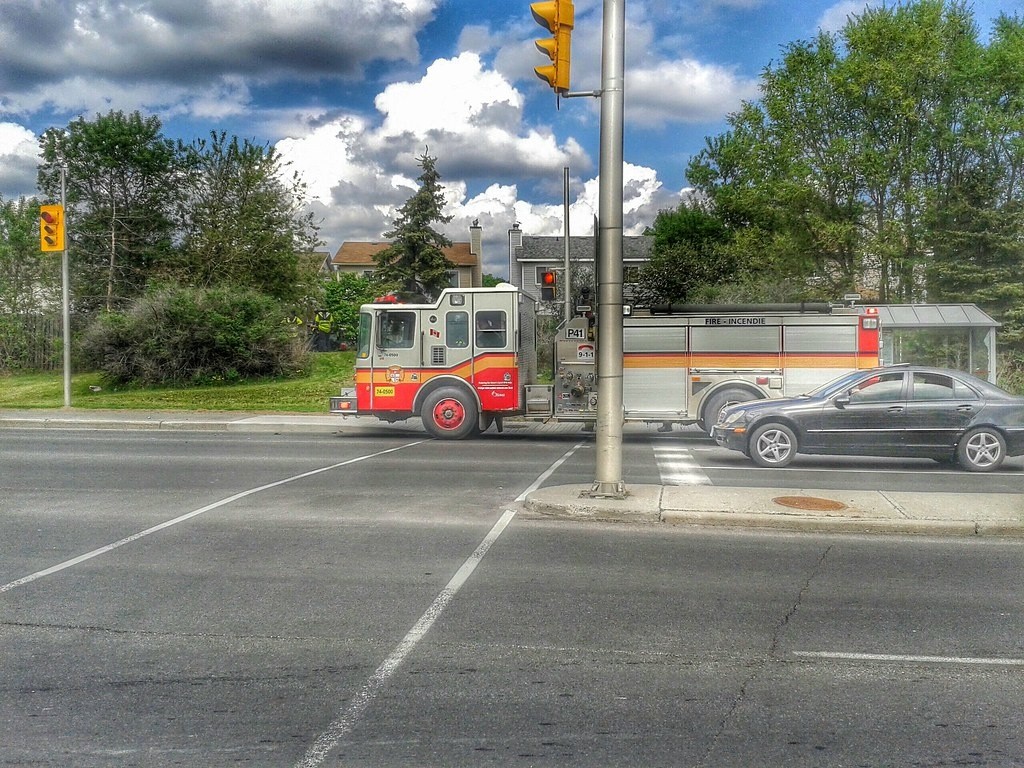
852, 375, 901, 400
310, 308, 334, 352
477, 319, 503, 346
280, 310, 303, 326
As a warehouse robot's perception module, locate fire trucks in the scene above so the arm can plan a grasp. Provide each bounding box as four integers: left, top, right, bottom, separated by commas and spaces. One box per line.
329, 283, 881, 440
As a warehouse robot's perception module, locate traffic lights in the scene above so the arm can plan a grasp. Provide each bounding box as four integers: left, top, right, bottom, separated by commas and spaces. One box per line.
40, 206, 67, 254
541, 271, 556, 301
532, 1, 574, 93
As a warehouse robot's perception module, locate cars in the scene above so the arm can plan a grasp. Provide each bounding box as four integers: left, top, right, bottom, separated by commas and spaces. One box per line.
710, 361, 1024, 471
